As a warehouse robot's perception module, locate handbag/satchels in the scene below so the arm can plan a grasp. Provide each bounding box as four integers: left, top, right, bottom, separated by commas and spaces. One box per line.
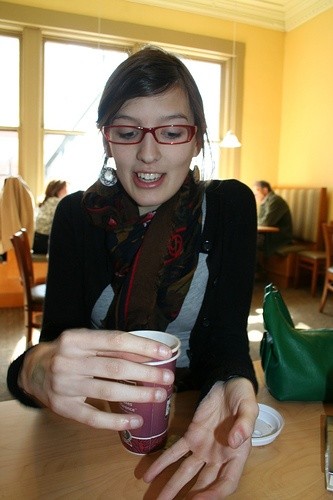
259, 283, 333, 401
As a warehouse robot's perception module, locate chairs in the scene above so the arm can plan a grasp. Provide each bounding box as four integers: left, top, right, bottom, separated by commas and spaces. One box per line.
10, 228, 46, 342
294, 220, 333, 313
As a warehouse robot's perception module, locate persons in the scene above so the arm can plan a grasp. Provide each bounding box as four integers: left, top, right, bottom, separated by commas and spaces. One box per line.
33, 180, 67, 255
256, 180, 293, 272
6, 44, 259, 500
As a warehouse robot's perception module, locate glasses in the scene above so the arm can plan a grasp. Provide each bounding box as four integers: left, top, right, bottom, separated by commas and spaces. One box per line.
100, 124, 198, 145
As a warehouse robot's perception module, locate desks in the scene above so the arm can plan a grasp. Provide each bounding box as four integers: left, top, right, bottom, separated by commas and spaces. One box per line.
0, 360, 332, 500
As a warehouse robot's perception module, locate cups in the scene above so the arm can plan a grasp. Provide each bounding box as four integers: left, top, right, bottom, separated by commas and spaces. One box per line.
106, 329, 184, 457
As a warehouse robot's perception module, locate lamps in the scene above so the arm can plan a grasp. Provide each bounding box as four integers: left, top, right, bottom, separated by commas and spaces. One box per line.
218, 22, 242, 147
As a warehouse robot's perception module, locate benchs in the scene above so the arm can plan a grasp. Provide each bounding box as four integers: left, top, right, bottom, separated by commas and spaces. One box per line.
270, 185, 327, 290
0, 177, 49, 308
